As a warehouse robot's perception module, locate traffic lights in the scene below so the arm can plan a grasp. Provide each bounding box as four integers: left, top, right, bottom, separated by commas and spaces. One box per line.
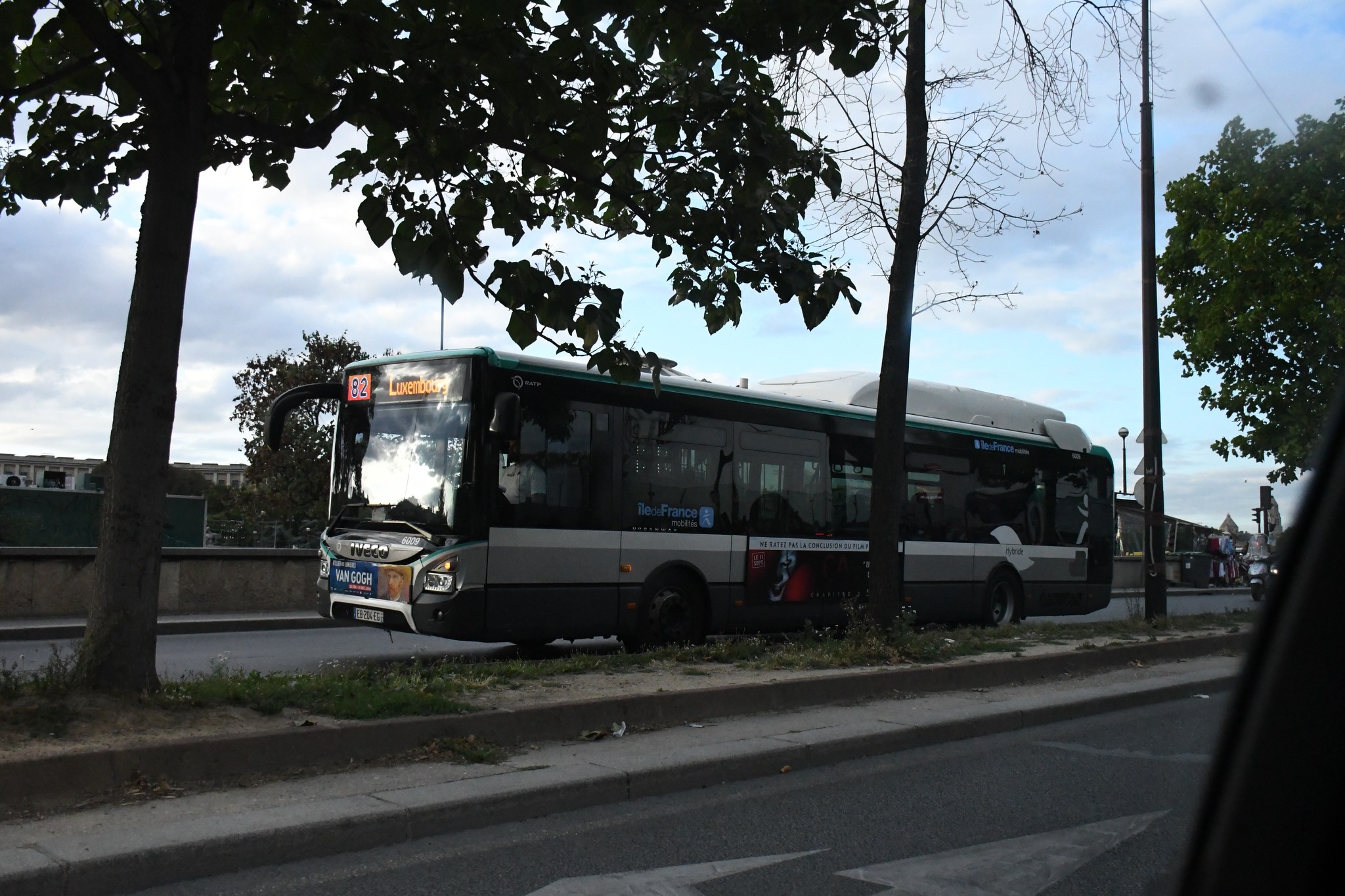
1251, 508, 1260, 523
1260, 486, 1274, 510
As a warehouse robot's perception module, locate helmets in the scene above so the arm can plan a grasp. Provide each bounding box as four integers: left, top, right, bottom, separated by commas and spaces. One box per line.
1257, 534, 1268, 545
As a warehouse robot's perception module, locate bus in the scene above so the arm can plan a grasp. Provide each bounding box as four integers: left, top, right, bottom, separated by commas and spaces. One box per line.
264, 349, 1116, 662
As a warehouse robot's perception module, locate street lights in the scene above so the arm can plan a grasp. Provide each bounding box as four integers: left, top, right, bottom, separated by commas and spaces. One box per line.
1118, 427, 1129, 493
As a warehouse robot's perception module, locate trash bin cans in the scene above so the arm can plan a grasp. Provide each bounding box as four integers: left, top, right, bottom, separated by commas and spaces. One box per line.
1180, 551, 1212, 588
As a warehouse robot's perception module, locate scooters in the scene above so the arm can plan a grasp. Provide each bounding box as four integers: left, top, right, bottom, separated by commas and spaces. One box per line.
1237, 534, 1277, 602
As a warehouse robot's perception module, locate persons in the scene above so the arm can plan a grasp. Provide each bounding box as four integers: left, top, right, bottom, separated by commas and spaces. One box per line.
1242, 533, 1275, 560
499, 441, 547, 505
